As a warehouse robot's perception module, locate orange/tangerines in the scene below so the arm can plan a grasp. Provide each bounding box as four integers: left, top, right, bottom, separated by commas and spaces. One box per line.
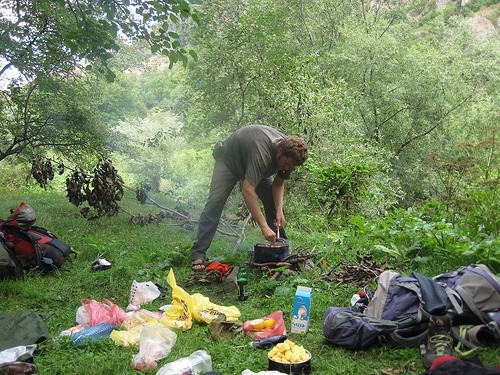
250, 319, 275, 329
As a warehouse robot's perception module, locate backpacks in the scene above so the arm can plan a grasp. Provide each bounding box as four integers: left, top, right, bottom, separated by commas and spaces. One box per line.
0, 203, 71, 275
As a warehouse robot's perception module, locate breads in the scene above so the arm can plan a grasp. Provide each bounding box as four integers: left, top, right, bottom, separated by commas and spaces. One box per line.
268, 339, 310, 362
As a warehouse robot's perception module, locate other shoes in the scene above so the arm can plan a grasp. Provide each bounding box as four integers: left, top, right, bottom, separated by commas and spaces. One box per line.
419, 330, 452, 366
450, 325, 493, 347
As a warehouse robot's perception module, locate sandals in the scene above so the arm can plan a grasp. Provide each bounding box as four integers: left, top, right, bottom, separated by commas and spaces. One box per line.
191, 258, 206, 271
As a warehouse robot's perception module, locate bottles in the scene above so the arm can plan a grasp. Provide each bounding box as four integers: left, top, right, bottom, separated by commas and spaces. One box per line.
71, 322, 114, 345
155, 349, 213, 374
51, 324, 86, 342
237, 268, 248, 301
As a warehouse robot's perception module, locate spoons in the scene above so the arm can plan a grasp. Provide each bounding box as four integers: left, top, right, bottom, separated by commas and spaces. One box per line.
275, 223, 286, 243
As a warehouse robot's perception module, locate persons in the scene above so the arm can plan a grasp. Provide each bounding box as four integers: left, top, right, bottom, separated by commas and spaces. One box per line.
189, 124, 309, 271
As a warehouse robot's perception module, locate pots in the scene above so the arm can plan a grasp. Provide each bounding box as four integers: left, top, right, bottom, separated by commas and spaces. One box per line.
254, 241, 289, 264
267, 349, 312, 375
